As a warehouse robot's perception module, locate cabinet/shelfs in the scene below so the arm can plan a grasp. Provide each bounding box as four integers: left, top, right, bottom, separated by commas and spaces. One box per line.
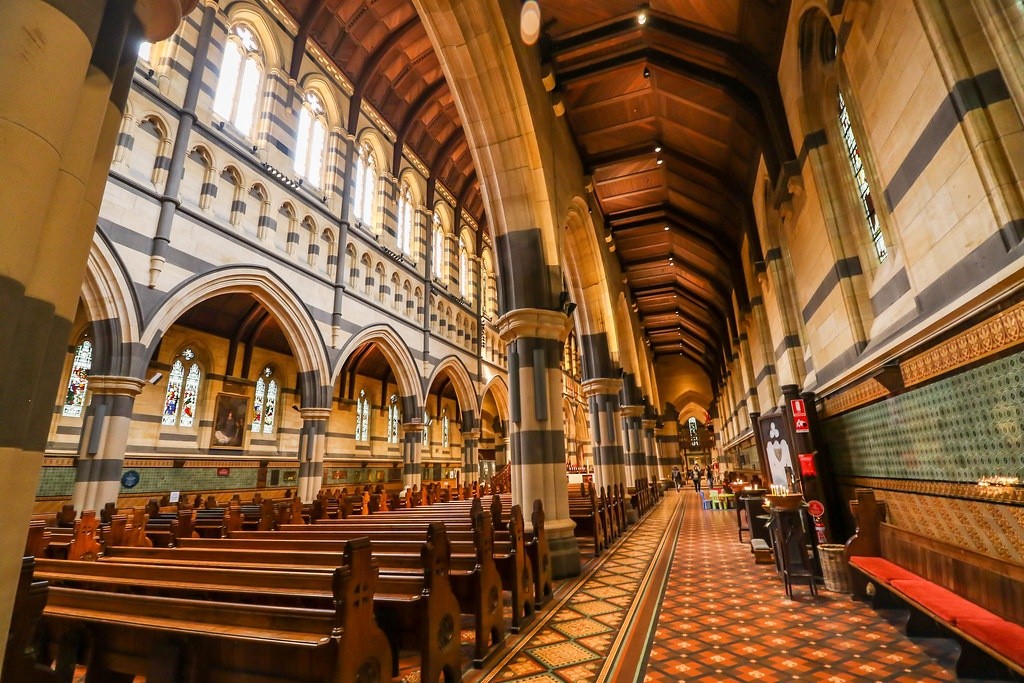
740, 496, 766, 539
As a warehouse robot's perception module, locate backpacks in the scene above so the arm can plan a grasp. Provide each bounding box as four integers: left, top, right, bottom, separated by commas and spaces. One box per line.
693, 470, 699, 481
673, 470, 680, 479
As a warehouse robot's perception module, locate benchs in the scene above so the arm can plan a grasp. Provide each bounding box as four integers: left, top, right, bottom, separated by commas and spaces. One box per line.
849, 555, 1024, 682
750, 538, 774, 564
2, 475, 671, 683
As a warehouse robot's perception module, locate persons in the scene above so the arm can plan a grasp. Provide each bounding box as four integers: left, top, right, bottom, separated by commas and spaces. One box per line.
691, 467, 701, 494
707, 467, 714, 490
672, 467, 681, 493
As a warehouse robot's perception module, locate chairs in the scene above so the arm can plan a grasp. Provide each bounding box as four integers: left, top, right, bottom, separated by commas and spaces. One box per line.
709, 490, 723, 510
700, 490, 712, 510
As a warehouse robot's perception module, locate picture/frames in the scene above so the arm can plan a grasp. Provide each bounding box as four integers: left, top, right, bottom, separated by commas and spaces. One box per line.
209, 391, 251, 451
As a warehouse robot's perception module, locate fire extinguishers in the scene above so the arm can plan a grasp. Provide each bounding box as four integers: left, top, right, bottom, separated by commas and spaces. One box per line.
815, 517, 827, 543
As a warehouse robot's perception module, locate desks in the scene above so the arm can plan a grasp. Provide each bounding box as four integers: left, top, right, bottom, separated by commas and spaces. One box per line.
734, 488, 768, 543
769, 508, 818, 602
730, 480, 750, 493
719, 493, 735, 509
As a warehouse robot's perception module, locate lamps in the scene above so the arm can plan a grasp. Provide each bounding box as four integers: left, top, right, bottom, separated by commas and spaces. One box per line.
656, 157, 663, 164
644, 67, 651, 78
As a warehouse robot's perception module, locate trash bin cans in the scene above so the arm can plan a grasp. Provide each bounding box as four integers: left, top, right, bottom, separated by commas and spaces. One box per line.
817, 544, 849, 593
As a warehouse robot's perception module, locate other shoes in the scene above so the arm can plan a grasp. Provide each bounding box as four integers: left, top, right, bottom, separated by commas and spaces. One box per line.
696, 492, 699, 495
677, 489, 680, 493
